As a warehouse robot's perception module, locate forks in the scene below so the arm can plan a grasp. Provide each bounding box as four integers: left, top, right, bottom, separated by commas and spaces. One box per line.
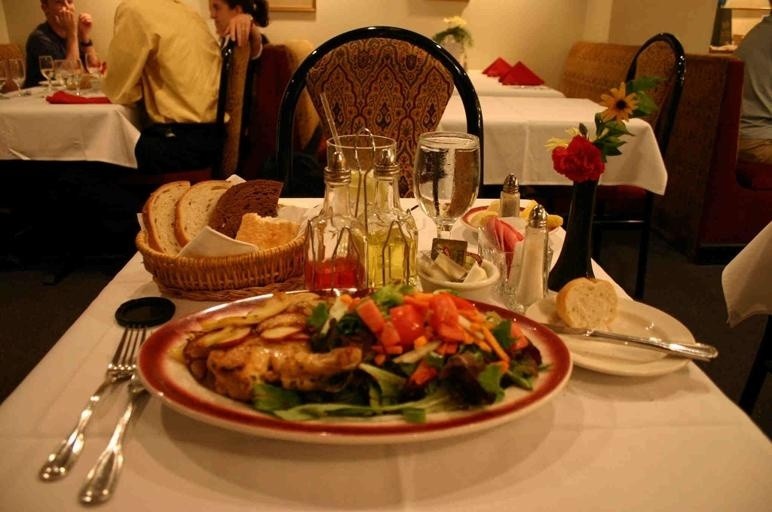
39, 321, 148, 483
78, 373, 152, 505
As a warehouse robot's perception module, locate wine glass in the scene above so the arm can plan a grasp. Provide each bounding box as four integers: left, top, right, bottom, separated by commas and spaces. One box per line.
410, 130, 483, 240
1, 51, 103, 104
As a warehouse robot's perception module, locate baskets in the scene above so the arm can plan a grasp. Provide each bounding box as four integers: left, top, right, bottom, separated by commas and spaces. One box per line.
135, 205, 306, 301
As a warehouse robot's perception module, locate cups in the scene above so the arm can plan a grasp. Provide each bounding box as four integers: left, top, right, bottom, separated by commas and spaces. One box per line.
326, 132, 398, 206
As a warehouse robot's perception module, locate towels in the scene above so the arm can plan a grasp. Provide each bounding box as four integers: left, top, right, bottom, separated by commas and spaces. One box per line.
498, 60, 547, 88
45, 89, 112, 105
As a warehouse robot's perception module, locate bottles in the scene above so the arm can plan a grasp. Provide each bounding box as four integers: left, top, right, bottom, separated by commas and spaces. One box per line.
510, 204, 548, 307
301, 149, 365, 292
498, 170, 522, 221
357, 149, 420, 291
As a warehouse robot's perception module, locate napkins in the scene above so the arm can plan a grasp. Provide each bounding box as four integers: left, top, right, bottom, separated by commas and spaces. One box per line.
481, 55, 513, 79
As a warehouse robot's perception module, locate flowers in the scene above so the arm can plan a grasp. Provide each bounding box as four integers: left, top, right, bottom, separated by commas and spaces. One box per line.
544, 72, 673, 186
431, 15, 479, 52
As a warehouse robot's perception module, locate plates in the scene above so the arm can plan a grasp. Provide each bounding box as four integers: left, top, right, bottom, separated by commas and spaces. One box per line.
136, 288, 570, 445
461, 204, 560, 237
518, 290, 699, 378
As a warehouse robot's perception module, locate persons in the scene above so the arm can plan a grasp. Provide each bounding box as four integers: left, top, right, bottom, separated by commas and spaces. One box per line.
207, 0, 276, 130
102, 0, 222, 178
23, 1, 99, 90
733, 0, 772, 167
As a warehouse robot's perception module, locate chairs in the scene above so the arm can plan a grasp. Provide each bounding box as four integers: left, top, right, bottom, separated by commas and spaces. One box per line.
126, 31, 253, 189
275, 25, 484, 196
561, 32, 687, 289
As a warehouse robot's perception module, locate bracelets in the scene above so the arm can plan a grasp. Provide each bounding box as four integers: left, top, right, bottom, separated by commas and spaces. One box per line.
80, 40, 93, 46
247, 14, 254, 25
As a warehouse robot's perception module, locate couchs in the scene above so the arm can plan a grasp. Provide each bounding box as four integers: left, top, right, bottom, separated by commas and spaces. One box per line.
554, 38, 770, 264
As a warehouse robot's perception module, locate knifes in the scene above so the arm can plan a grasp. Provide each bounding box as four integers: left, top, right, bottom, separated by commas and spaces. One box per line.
539, 318, 716, 358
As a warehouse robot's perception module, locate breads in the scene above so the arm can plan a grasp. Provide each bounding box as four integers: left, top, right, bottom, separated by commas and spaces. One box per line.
143, 180, 299, 256
556, 278, 617, 330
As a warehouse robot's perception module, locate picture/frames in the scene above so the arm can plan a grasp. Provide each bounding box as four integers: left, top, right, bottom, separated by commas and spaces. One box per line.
208, 1, 317, 19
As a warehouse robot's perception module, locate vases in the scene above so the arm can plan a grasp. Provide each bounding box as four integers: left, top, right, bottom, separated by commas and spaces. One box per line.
544, 173, 609, 293
